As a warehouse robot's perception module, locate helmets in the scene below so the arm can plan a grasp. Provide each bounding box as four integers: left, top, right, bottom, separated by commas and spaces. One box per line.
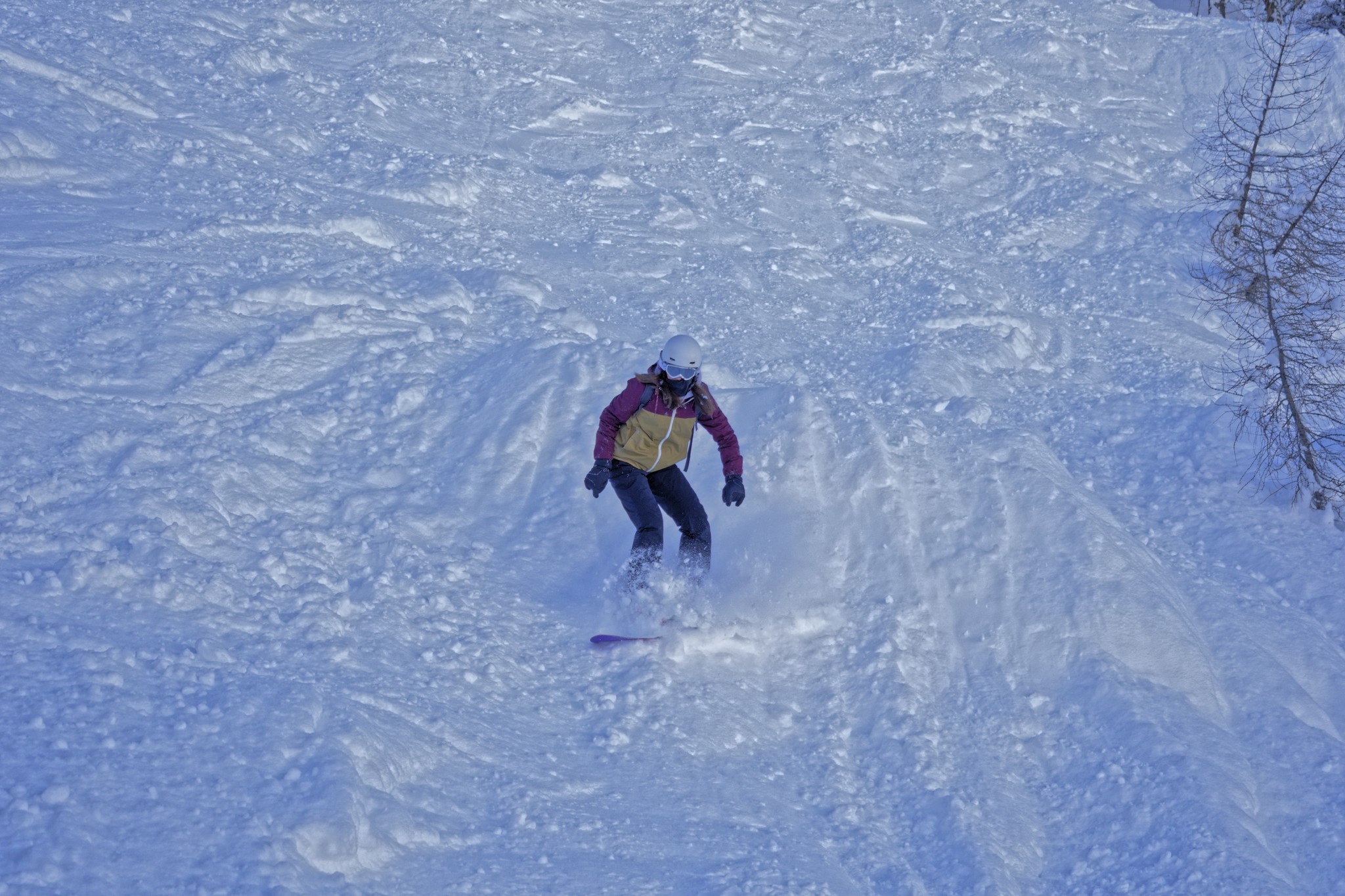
658, 335, 704, 372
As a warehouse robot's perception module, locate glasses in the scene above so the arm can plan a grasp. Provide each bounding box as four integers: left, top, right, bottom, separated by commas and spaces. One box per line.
664, 362, 698, 381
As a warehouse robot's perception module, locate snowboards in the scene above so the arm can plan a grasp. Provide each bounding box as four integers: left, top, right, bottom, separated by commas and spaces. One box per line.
590, 625, 697, 643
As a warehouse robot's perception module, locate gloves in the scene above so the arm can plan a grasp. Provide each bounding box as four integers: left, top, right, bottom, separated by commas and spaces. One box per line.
584, 461, 611, 499
721, 474, 745, 508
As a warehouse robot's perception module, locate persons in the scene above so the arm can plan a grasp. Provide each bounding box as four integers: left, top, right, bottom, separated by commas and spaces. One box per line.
584, 332, 746, 622
